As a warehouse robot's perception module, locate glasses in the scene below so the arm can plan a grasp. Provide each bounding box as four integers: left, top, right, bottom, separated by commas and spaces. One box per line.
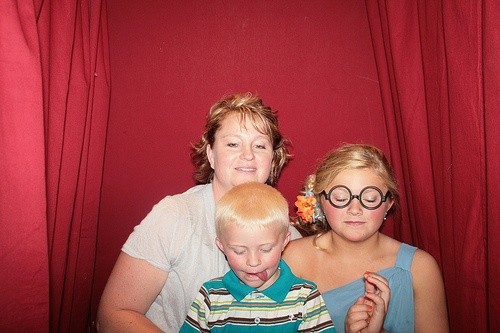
322, 185, 390, 210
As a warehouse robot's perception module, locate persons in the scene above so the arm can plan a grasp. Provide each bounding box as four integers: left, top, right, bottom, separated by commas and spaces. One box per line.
177, 181, 336, 333
96, 92, 303, 333
281, 142, 450, 333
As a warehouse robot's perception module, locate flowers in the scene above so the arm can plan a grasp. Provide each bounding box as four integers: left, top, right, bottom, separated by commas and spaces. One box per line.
295, 190, 320, 224
